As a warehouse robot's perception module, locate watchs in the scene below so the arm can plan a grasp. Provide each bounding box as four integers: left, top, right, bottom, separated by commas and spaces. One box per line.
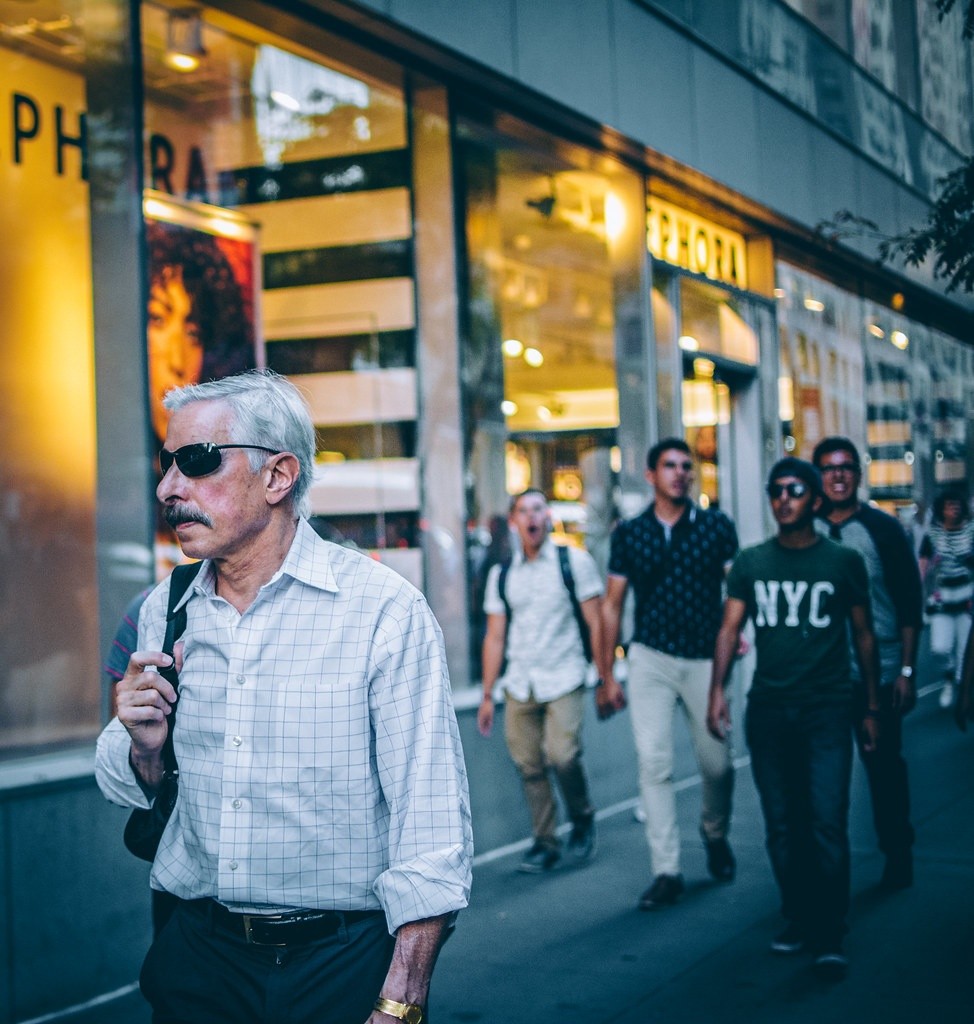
900, 665, 916, 678
373, 996, 424, 1024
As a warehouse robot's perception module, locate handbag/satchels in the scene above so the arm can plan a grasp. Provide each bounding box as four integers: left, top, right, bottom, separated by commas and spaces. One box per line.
123, 561, 201, 862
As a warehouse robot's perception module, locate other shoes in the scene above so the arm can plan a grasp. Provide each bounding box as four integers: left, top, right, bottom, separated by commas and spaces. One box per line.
520, 844, 562, 874
940, 682, 953, 706
772, 926, 814, 952
640, 873, 683, 909
817, 937, 848, 975
555, 831, 596, 868
882, 851, 915, 889
701, 827, 737, 881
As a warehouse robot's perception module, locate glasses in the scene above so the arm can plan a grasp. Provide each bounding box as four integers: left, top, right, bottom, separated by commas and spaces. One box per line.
159, 441, 279, 477
766, 483, 809, 497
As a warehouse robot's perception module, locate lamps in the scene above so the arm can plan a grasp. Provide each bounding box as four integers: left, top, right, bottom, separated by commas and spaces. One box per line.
524, 196, 556, 218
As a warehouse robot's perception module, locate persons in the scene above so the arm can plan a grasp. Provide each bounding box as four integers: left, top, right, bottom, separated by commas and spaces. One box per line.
599, 437, 748, 911
95, 368, 476, 1024
142, 217, 259, 586
813, 438, 973, 892
704, 458, 881, 972
476, 489, 618, 874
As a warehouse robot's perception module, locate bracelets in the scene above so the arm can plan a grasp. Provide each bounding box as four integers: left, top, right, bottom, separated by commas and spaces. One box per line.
868, 709, 882, 718
596, 678, 605, 688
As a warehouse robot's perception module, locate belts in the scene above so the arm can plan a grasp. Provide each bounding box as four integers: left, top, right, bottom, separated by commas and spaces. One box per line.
194, 900, 381, 950
